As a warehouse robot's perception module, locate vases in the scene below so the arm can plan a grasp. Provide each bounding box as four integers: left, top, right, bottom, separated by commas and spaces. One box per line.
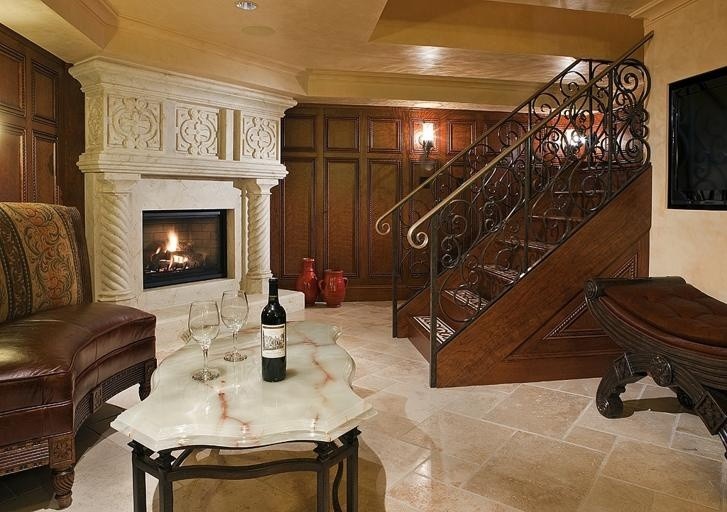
318, 269, 349, 308
296, 257, 318, 306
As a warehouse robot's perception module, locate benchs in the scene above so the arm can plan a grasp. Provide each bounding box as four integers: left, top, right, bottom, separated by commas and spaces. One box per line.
583, 276, 727, 460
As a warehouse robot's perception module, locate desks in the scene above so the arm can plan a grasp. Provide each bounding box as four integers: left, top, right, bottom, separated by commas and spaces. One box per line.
110, 320, 376, 512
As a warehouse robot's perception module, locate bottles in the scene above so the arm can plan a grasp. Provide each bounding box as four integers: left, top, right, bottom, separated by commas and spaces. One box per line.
262, 278, 288, 382
297, 257, 349, 309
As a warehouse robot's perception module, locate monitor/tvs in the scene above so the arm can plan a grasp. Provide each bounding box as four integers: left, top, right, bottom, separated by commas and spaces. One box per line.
666, 64, 727, 211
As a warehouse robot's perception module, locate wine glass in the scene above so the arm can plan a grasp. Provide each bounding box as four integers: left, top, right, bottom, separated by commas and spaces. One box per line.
189, 290, 250, 383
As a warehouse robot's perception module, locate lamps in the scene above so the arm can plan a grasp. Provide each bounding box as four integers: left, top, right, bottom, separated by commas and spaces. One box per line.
418, 123, 435, 161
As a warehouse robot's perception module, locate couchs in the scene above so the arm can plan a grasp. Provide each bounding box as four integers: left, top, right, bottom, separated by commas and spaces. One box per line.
0, 202, 157, 508
